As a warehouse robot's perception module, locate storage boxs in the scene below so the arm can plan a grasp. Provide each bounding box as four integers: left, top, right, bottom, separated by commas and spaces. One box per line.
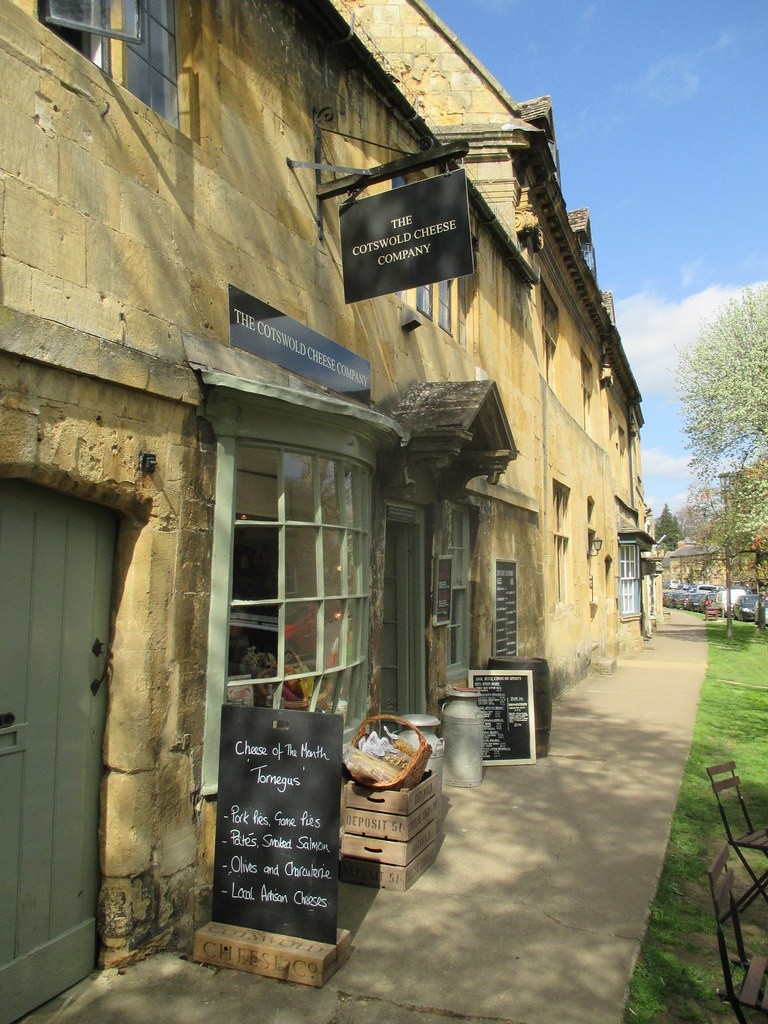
339, 769, 441, 891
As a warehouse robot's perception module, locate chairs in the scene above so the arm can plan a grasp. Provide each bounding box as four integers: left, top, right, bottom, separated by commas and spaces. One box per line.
707, 842, 768, 1024
705, 761, 768, 923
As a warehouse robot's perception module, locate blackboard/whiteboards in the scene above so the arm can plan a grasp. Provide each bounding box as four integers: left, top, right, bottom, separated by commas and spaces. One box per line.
468, 669, 537, 766
492, 558, 519, 658
211, 704, 344, 946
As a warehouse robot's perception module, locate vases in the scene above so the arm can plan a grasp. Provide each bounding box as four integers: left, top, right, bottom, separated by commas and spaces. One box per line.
705, 606, 718, 622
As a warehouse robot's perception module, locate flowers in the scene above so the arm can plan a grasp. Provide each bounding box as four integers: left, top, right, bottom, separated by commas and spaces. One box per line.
704, 599, 715, 607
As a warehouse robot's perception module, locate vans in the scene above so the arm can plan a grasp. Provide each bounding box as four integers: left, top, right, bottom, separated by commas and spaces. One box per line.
668, 579, 680, 590
716, 589, 746, 617
696, 585, 726, 593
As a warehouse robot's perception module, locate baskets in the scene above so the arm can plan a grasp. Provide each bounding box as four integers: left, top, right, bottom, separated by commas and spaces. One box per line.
257, 648, 332, 710
349, 714, 432, 791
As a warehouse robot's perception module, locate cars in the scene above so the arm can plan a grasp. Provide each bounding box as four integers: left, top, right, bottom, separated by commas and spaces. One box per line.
686, 593, 705, 612
665, 592, 682, 608
662, 579, 671, 589
676, 593, 691, 609
733, 595, 765, 622
682, 584, 691, 590
754, 599, 768, 626
662, 591, 670, 606
677, 584, 683, 590
689, 585, 697, 593
698, 593, 722, 613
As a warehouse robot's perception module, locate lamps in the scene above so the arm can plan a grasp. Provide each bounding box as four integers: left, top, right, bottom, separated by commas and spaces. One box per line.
585, 536, 603, 559
